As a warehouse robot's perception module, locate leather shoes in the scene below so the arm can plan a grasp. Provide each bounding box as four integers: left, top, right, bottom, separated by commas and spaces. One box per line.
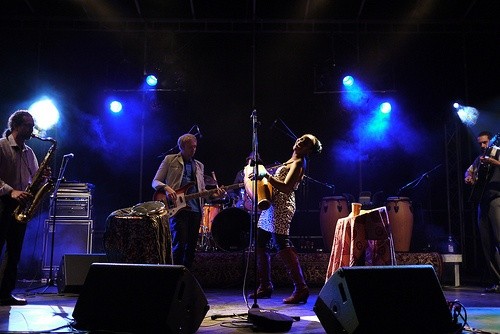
0, 294, 28, 304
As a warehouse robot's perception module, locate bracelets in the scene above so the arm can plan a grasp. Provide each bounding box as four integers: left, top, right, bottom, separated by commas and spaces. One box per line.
268, 175, 272, 181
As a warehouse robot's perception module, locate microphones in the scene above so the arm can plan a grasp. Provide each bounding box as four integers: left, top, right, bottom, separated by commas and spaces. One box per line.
212, 172, 219, 189
271, 118, 280, 127
250, 110, 256, 118
196, 125, 203, 138
64, 153, 75, 158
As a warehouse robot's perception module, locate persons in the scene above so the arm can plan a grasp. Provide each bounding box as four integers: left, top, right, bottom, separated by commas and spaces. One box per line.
248, 134, 322, 304
152, 134, 227, 271
464, 132, 500, 292
0, 111, 52, 304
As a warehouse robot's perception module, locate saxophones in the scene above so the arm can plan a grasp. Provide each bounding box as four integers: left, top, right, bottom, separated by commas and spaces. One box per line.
11, 133, 55, 226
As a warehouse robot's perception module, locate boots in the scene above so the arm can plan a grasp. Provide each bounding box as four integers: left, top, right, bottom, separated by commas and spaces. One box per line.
278, 248, 309, 304
248, 248, 273, 299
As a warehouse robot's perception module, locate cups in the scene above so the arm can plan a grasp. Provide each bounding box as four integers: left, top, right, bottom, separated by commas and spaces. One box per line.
351, 202, 362, 216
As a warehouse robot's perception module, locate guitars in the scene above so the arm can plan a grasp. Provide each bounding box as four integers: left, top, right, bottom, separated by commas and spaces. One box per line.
153, 182, 244, 219
469, 134, 500, 201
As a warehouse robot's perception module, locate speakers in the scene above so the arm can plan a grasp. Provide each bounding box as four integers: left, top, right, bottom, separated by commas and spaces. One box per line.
313, 265, 457, 334
43, 219, 210, 334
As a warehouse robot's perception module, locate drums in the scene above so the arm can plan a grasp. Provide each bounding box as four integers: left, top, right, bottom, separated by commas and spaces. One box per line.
319, 196, 350, 253
243, 163, 272, 210
211, 207, 254, 252
386, 196, 414, 253
197, 202, 222, 235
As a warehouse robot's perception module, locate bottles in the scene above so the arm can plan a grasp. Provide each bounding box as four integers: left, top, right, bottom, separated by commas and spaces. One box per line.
448, 234, 454, 254
